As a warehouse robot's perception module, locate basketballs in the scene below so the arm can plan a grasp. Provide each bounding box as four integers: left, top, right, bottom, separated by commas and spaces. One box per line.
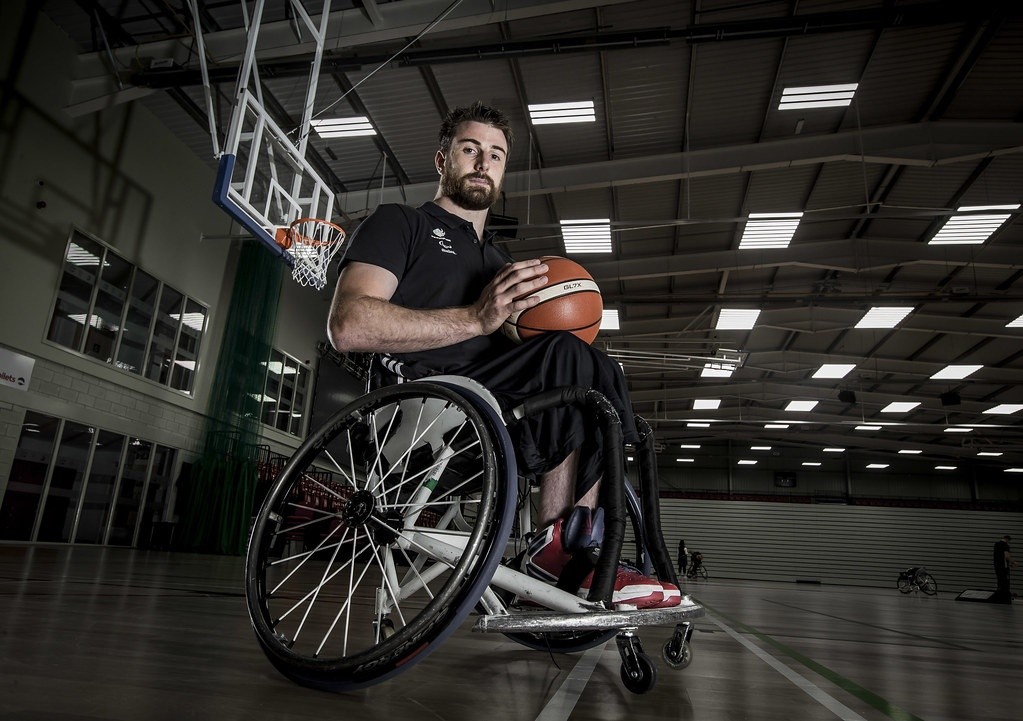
500, 256, 603, 346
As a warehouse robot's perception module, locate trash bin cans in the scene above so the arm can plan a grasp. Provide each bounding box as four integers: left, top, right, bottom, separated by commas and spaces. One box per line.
148, 521, 176, 552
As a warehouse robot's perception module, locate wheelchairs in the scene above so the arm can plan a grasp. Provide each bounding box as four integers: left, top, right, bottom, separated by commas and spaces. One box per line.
242, 337, 707, 695
686, 562, 708, 581
896, 571, 938, 596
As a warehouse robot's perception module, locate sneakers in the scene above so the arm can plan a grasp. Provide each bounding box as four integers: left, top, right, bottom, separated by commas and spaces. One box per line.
592, 507, 681, 607
519, 517, 664, 610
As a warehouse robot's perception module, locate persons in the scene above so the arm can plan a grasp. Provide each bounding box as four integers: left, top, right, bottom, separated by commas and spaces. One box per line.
687, 550, 703, 580
677, 541, 688, 577
993, 536, 1018, 604
325, 104, 680, 613
